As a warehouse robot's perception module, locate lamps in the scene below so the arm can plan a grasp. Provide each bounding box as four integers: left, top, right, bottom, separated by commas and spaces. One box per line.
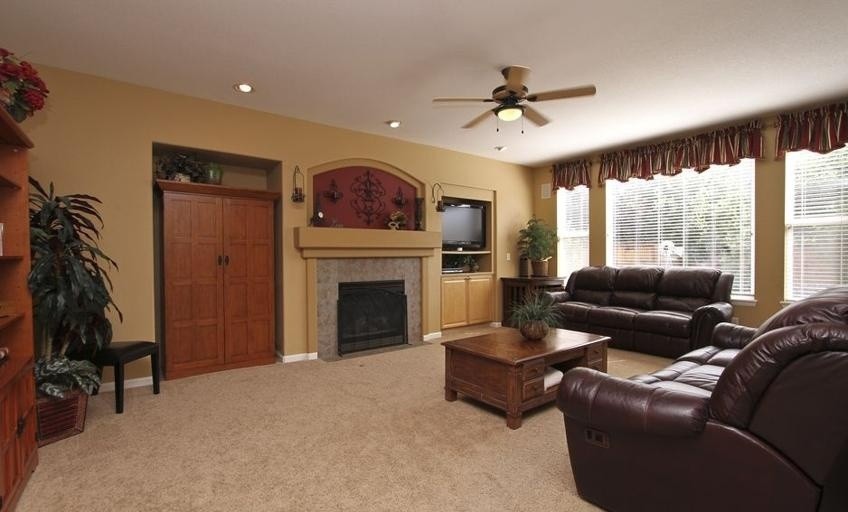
290, 163, 305, 203
429, 183, 446, 212
491, 105, 524, 123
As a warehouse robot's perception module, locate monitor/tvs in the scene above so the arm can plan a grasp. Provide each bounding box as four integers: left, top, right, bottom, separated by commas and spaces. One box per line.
442, 202, 486, 248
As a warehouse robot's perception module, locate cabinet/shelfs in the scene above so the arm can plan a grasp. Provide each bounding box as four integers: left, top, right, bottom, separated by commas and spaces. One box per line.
441, 272, 493, 331
0, 108, 41, 512
156, 178, 280, 381
500, 277, 566, 327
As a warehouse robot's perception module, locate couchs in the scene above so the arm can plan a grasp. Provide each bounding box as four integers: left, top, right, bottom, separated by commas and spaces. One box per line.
556, 285, 846, 511
537, 265, 734, 362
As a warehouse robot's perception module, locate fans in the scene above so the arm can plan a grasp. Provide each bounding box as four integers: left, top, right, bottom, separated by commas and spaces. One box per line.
431, 65, 597, 130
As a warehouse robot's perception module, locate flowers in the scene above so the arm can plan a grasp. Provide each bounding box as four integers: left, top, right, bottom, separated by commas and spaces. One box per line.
0, 47, 47, 120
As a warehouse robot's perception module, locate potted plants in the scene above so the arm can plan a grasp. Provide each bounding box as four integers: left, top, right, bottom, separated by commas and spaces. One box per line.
26, 177, 124, 447
159, 150, 207, 182
508, 287, 566, 340
516, 218, 557, 276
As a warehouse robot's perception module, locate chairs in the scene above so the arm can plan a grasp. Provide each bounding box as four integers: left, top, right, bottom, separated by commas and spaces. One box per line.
91, 327, 158, 414
